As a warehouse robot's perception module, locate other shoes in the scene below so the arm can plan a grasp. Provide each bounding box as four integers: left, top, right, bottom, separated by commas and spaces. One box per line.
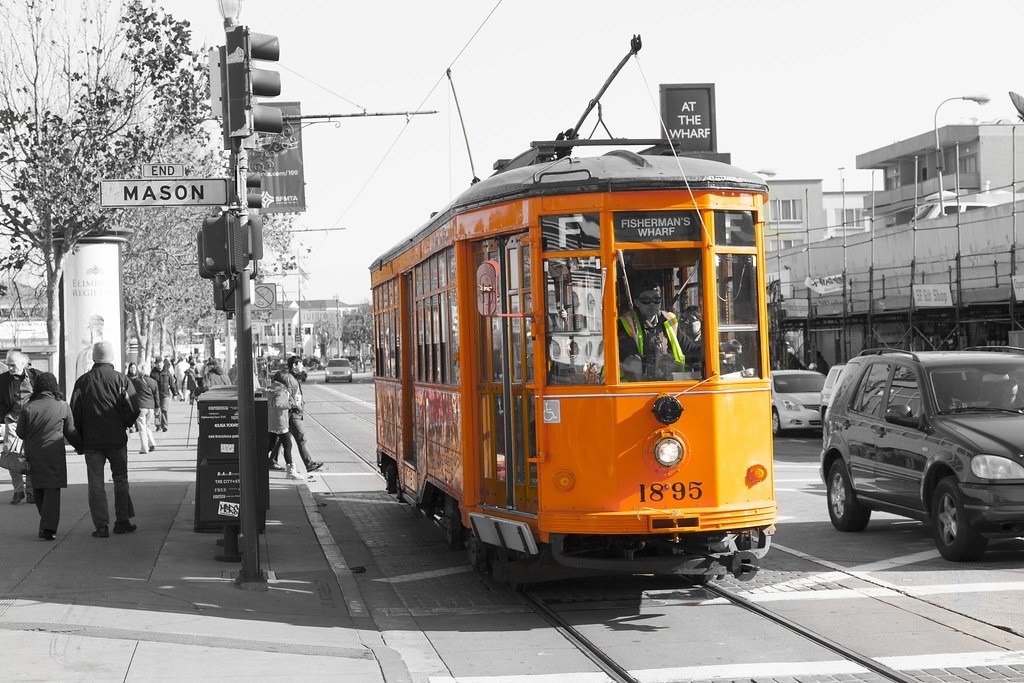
139, 451, 146, 454
10, 490, 25, 504
92, 524, 109, 537
39, 530, 56, 540
113, 520, 137, 533
269, 461, 286, 471
307, 461, 323, 472
149, 445, 154, 451
163, 428, 167, 432
27, 494, 35, 503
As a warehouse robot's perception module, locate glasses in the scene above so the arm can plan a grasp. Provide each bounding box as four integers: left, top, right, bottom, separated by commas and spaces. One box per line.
638, 296, 664, 304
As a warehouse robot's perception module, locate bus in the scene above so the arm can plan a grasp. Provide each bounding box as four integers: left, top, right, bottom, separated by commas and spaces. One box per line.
367, 32, 782, 596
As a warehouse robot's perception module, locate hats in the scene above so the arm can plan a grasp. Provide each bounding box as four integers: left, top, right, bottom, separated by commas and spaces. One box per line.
154, 357, 162, 363
206, 361, 217, 365
92, 341, 114, 363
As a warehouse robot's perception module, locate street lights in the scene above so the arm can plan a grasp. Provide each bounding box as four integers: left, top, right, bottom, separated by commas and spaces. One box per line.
933, 95, 991, 217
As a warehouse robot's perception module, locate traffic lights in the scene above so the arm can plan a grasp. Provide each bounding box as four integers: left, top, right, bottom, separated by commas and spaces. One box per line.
245, 24, 284, 134
246, 172, 263, 210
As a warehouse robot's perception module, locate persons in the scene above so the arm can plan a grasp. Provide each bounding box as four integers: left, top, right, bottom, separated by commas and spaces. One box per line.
126, 362, 140, 433
150, 355, 238, 432
804, 351, 830, 376
989, 374, 1024, 406
938, 331, 958, 351
780, 352, 807, 370
69, 342, 141, 537
16, 372, 86, 540
269, 356, 324, 472
133, 362, 160, 454
616, 280, 742, 377
680, 306, 701, 342
0, 350, 46, 504
265, 371, 306, 480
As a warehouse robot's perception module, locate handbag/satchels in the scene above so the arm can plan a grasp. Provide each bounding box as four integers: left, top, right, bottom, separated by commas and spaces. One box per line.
0, 436, 30, 474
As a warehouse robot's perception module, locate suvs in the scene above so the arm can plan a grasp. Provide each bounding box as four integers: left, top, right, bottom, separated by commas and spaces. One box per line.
818, 344, 1024, 566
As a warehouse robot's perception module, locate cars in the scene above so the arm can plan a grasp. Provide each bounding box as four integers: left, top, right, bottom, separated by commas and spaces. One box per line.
769, 368, 827, 438
324, 358, 353, 384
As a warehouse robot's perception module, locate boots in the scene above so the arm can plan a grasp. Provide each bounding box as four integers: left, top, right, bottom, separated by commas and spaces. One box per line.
286, 462, 304, 479
